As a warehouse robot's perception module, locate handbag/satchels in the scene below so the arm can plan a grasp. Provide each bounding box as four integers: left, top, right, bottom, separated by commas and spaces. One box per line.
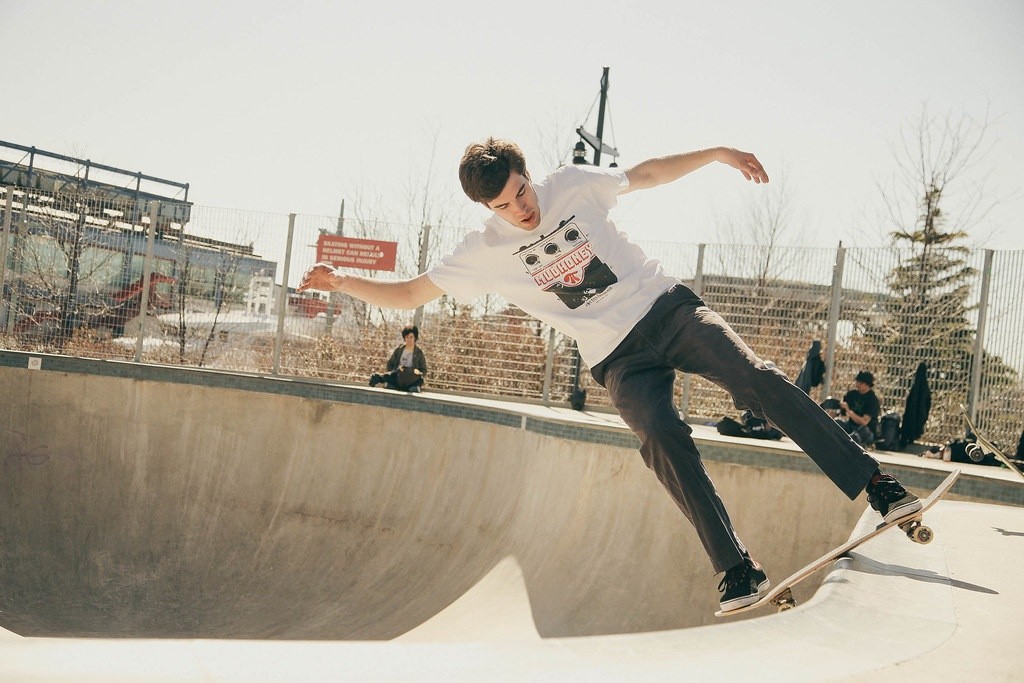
716, 416, 783, 440
878, 413, 907, 452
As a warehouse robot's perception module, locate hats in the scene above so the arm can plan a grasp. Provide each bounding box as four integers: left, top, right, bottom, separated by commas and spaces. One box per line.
855, 370, 874, 385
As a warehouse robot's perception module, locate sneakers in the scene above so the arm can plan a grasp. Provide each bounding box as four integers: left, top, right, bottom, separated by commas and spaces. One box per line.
866, 474, 922, 524
713, 561, 770, 611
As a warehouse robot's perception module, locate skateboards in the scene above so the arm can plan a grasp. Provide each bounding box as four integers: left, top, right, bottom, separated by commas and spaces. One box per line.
713, 466, 963, 618
958, 403, 1024, 480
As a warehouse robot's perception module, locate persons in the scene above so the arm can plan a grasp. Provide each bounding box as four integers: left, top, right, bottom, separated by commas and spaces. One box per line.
295, 138, 923, 611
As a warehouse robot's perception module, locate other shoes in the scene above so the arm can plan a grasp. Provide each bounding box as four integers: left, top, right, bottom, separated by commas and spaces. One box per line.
861, 443, 876, 450
